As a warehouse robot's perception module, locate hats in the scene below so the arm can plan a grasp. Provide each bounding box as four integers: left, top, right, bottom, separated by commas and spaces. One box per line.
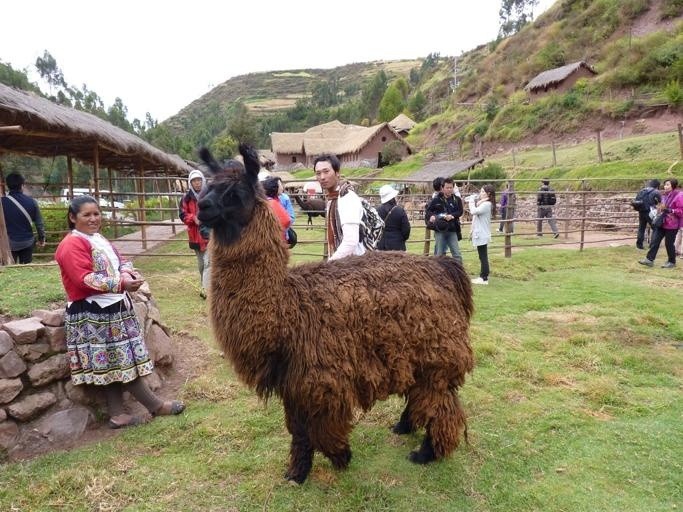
190, 172, 202, 181
379, 185, 400, 204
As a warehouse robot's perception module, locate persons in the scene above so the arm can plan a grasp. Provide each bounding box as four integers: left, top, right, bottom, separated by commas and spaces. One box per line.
2, 172, 45, 264
468, 185, 495, 285
265, 174, 296, 240
313, 153, 366, 263
497, 183, 514, 232
374, 185, 410, 251
535, 177, 559, 238
180, 169, 212, 299
52, 195, 187, 428
637, 179, 682, 267
262, 179, 287, 247
634, 180, 660, 249
427, 178, 462, 265
432, 177, 461, 200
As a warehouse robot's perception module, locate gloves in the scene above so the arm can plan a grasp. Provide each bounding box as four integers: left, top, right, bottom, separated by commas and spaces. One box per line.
663, 208, 672, 213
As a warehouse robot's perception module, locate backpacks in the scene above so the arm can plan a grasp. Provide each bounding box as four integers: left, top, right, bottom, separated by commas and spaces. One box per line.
335, 189, 386, 251
544, 189, 556, 205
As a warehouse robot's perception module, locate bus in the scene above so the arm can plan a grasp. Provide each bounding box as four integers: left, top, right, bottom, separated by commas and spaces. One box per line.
58, 187, 130, 210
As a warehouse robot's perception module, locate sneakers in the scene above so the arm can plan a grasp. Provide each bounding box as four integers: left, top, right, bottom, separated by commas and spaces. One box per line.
661, 262, 675, 268
470, 276, 489, 285
553, 233, 559, 238
151, 400, 186, 416
638, 259, 653, 267
109, 415, 149, 428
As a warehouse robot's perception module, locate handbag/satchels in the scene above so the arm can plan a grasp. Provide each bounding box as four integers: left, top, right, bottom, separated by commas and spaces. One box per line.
425, 203, 452, 233
631, 199, 645, 211
289, 227, 297, 249
652, 212, 666, 228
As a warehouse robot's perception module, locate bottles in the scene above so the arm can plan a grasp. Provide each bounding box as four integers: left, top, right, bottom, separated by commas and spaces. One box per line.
465, 195, 479, 202
